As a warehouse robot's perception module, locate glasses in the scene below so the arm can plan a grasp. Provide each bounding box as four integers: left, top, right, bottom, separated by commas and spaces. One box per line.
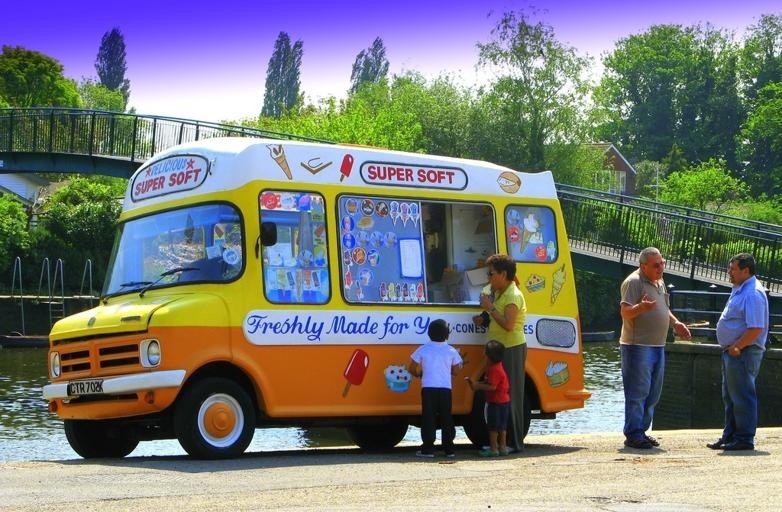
487, 271, 498, 275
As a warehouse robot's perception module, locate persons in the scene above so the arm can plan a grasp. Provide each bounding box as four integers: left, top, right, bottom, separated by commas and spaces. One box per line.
471, 254, 527, 452
469, 340, 511, 458
407, 319, 464, 460
618, 246, 692, 450
706, 252, 770, 451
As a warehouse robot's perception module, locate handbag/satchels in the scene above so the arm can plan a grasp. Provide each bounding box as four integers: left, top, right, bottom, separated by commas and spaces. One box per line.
464, 266, 489, 301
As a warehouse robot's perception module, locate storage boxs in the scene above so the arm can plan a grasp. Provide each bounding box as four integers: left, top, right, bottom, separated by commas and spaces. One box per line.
438, 267, 489, 303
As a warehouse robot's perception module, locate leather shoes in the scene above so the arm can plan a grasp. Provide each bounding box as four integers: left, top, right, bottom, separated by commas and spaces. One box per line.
707, 439, 730, 449
720, 439, 754, 449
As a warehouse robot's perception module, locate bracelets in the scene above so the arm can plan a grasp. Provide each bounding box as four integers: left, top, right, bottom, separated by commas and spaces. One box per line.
487, 307, 496, 314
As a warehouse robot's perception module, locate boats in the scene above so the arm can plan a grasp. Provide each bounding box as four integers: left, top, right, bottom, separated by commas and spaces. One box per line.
581, 328, 616, 342
0, 330, 49, 349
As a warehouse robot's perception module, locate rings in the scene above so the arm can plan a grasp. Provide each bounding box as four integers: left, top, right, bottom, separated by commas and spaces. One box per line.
479, 294, 484, 296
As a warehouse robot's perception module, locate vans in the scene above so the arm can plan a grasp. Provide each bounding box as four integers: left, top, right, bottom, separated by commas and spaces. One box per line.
39, 136, 594, 458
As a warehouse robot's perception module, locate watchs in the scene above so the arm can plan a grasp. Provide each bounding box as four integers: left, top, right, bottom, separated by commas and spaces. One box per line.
733, 344, 742, 354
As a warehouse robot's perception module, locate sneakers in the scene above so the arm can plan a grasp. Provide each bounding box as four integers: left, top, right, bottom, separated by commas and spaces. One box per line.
479, 446, 514, 457
416, 450, 435, 458
443, 449, 455, 457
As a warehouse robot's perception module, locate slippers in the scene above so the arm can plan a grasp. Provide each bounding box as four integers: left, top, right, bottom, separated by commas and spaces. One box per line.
624, 436, 659, 449
